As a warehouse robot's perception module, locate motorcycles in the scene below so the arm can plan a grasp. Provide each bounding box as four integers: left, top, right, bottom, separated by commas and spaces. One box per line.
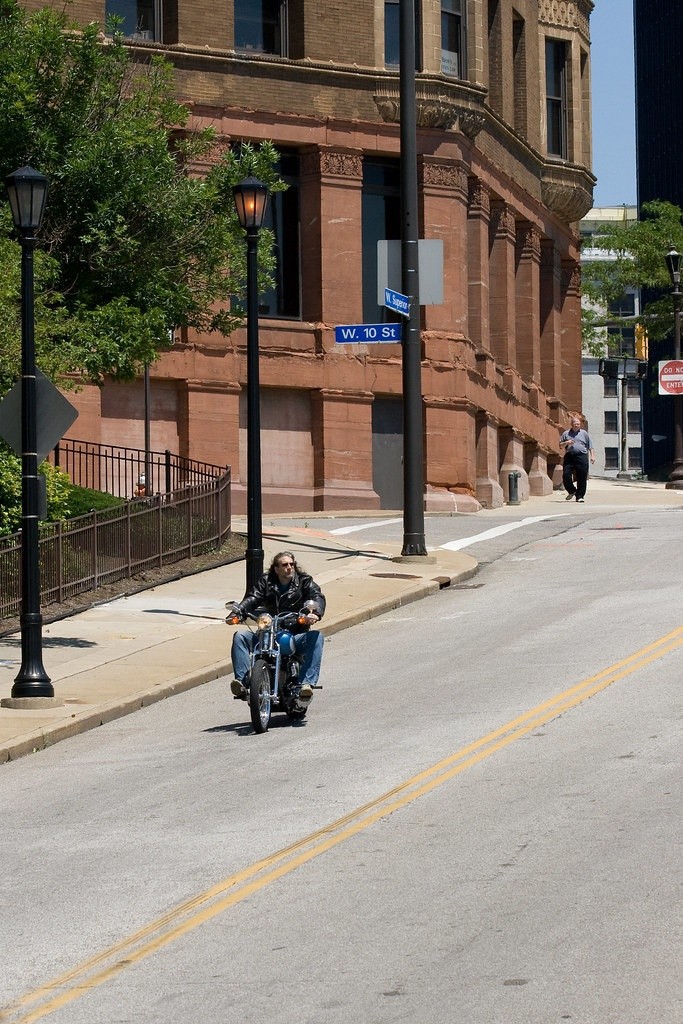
225, 601, 323, 734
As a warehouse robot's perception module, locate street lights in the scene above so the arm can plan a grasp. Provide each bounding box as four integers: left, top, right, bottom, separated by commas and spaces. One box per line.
232, 162, 270, 602
664, 244, 683, 490
6, 162, 55, 698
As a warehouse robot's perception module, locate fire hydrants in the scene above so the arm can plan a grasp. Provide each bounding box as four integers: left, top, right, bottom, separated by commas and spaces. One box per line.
508, 471, 522, 505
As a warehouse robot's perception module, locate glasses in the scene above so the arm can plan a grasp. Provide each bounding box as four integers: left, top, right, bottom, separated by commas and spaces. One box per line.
277, 562, 295, 567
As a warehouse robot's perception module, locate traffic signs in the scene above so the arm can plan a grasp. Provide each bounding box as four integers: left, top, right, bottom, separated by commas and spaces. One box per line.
384, 286, 409, 316
333, 323, 404, 343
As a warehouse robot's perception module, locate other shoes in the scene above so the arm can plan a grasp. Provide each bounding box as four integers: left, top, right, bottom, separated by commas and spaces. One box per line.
576, 497, 584, 502
231, 679, 249, 701
566, 489, 577, 500
300, 684, 313, 696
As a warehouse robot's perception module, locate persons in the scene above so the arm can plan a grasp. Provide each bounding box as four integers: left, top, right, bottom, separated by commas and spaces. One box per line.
559, 417, 596, 502
226, 552, 326, 701
140, 472, 152, 485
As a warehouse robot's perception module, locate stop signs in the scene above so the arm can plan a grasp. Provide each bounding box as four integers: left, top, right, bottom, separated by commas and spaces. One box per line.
658, 359, 683, 395
